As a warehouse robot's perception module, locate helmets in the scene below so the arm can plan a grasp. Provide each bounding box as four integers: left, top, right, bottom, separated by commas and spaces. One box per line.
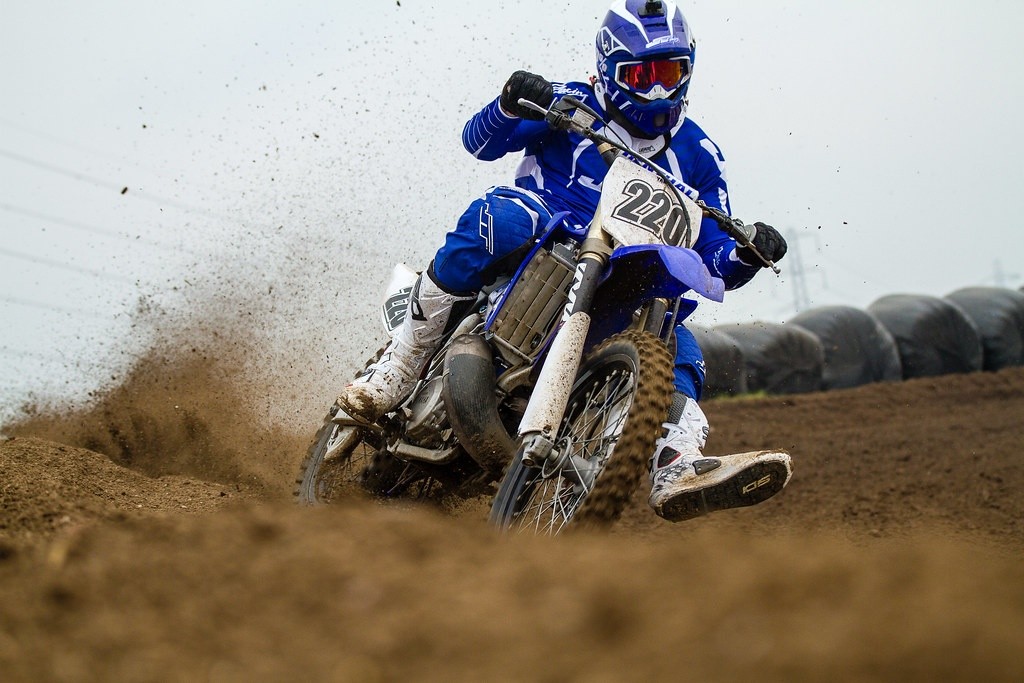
590, 0, 698, 123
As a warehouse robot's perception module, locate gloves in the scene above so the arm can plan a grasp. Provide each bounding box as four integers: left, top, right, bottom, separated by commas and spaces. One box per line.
498, 68, 554, 125
734, 217, 789, 268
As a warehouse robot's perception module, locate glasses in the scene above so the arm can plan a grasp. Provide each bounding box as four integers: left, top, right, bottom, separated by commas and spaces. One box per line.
620, 60, 688, 90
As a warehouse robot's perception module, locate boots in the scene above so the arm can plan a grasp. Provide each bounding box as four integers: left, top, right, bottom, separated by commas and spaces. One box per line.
333, 261, 479, 427
644, 392, 799, 522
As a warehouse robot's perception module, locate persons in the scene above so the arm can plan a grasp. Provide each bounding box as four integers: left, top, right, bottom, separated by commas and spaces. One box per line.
345, 0, 794, 520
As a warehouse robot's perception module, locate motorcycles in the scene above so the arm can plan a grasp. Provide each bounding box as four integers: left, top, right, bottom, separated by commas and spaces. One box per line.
291, 82, 783, 539
